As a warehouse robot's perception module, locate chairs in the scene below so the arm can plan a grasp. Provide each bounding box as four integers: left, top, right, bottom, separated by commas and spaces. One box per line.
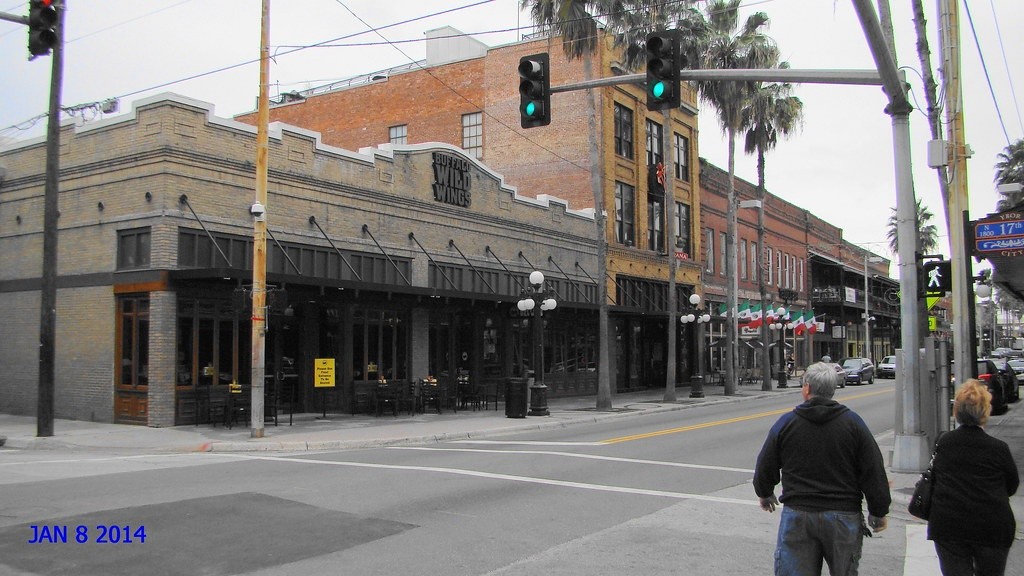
708, 366, 765, 386
350, 372, 502, 417
195, 380, 295, 431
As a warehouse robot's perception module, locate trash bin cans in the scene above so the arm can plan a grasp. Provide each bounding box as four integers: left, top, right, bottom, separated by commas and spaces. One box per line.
505, 377, 529, 418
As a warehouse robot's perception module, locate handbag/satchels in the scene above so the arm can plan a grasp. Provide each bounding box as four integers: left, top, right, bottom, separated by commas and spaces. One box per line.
908, 432, 948, 521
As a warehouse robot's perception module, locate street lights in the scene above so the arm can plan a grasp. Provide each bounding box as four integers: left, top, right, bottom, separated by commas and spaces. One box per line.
768, 306, 790, 388
517, 271, 558, 416
680, 294, 710, 398
861, 255, 883, 363
967, 268, 992, 379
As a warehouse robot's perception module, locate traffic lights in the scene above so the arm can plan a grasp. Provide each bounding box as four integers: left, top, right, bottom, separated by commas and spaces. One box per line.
646, 28, 681, 111
519, 53, 551, 130
924, 260, 952, 291
30, 0, 62, 57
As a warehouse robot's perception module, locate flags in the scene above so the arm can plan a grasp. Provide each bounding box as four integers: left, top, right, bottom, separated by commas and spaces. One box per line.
803, 310, 817, 335
766, 303, 774, 324
738, 300, 750, 318
718, 300, 734, 318
792, 309, 807, 335
750, 302, 762, 321
774, 305, 789, 325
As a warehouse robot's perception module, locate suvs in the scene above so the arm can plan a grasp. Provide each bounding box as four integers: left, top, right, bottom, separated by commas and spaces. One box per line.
839, 357, 875, 385
949, 360, 1006, 415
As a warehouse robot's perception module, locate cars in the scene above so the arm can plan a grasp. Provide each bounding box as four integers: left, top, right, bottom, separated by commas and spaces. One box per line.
992, 347, 1013, 359
800, 362, 847, 388
1008, 358, 1024, 384
876, 354, 895, 378
993, 360, 1019, 403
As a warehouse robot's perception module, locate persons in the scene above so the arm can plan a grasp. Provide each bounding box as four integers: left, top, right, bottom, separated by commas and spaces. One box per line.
787, 353, 794, 366
821, 352, 832, 362
753, 362, 892, 576
926, 377, 1020, 576
